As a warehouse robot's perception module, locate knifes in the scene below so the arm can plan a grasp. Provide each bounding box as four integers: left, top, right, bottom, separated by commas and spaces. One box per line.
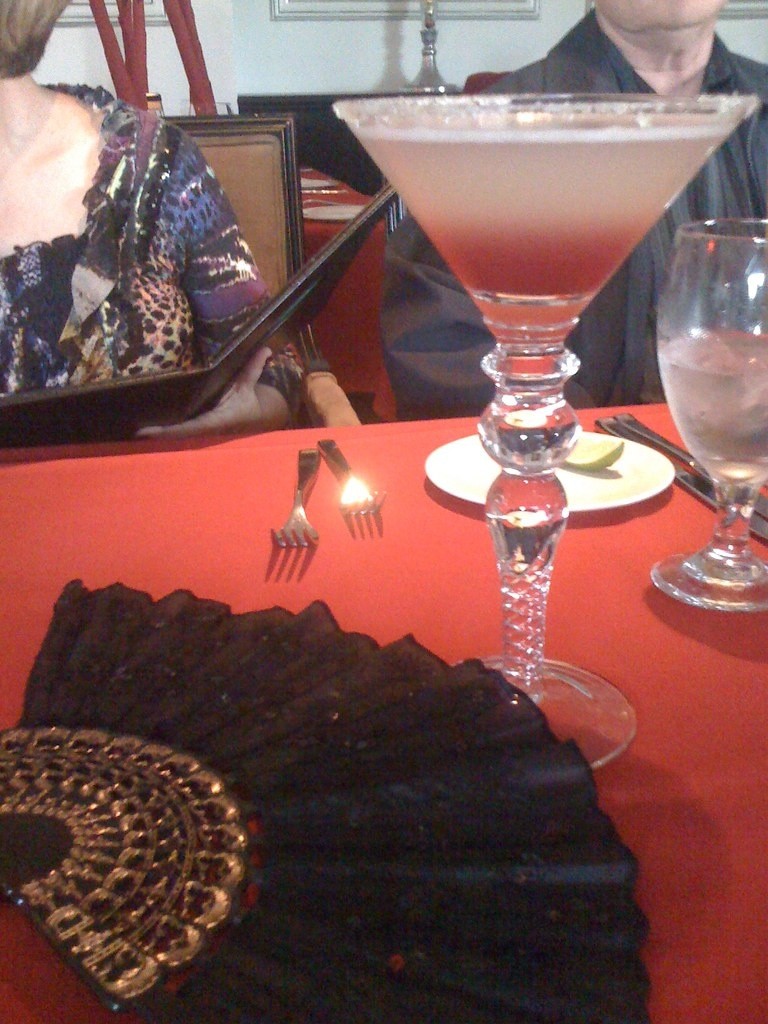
615, 414, 767, 521
595, 413, 768, 547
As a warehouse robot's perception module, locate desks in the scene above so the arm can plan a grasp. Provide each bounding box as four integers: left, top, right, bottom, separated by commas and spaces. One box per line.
295, 162, 387, 397
0, 402, 768, 1024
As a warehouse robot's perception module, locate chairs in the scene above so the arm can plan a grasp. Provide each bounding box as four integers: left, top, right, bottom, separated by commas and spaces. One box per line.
163, 116, 321, 424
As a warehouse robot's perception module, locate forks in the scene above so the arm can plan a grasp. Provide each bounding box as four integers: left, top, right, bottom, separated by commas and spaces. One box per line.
317, 438, 388, 520
269, 448, 320, 551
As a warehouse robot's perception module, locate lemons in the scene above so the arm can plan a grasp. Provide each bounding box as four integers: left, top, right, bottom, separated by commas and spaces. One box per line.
567, 439, 626, 470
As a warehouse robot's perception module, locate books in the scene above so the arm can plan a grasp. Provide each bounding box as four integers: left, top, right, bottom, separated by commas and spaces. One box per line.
2, 185, 397, 442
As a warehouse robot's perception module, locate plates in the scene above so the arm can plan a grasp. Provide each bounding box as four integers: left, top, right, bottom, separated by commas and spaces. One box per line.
422, 426, 676, 517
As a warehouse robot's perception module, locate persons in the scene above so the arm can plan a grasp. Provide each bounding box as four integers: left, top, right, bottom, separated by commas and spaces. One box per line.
379, 1, 768, 423
0, 0, 305, 444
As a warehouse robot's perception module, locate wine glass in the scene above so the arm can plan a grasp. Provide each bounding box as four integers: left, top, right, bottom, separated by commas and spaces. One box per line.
325, 76, 760, 768
651, 217, 768, 615
